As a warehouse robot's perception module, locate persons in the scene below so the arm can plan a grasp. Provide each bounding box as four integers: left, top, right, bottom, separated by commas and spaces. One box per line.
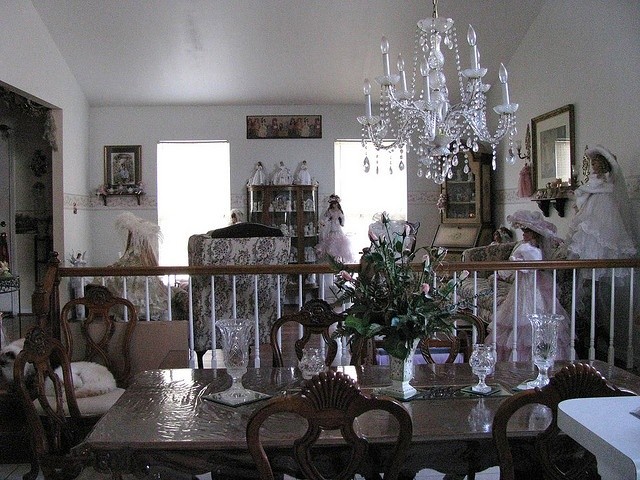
272, 161, 294, 185
252, 161, 269, 185
565, 144, 637, 288
247, 116, 320, 137
490, 225, 513, 245
482, 209, 581, 361
297, 160, 311, 185
228, 208, 243, 225
315, 194, 359, 264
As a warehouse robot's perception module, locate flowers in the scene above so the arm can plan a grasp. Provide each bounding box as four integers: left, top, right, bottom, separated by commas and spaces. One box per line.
324, 214, 492, 358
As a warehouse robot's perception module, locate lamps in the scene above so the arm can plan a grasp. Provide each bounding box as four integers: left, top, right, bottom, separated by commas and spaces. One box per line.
356, 0, 519, 185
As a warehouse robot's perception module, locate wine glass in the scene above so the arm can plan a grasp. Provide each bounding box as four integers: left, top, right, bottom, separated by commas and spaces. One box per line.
526, 313, 565, 389
215, 319, 254, 399
469, 343, 496, 393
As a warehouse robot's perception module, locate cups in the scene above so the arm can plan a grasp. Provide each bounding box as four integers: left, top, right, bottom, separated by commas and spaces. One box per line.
298, 348, 326, 380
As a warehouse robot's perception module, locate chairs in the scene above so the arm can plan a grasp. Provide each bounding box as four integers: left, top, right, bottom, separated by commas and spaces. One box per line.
31, 285, 137, 474
246, 370, 413, 480
270, 299, 376, 366
14, 326, 90, 480
492, 362, 637, 480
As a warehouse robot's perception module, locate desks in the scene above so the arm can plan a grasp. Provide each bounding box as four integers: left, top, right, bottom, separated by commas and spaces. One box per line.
86, 359, 640, 480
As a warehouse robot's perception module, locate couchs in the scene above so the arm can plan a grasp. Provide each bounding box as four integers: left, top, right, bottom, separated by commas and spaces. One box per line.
172, 220, 292, 369
455, 240, 574, 327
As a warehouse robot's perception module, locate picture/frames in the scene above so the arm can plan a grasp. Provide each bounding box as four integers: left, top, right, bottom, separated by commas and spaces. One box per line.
103, 145, 142, 191
530, 104, 578, 191
246, 115, 322, 139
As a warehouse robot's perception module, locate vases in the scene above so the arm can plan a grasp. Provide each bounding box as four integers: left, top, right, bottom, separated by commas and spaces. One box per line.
380, 336, 422, 401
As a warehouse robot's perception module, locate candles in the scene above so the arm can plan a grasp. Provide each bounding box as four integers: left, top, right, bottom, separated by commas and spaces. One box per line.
515, 138, 521, 151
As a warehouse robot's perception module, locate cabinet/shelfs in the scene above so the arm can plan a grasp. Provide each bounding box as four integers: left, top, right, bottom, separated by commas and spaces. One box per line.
246, 183, 321, 306
441, 140, 493, 227
